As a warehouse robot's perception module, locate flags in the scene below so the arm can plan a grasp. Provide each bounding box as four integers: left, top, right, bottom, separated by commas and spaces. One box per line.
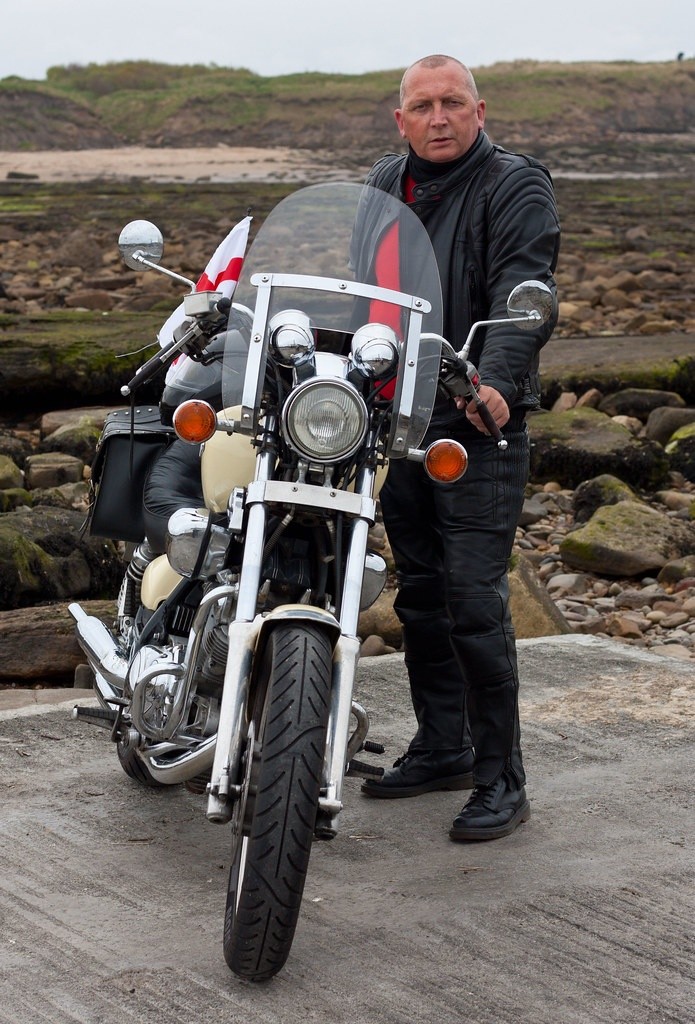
154, 215, 254, 385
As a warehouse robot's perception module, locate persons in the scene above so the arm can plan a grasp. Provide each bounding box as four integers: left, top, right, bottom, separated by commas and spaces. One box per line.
348, 52, 564, 845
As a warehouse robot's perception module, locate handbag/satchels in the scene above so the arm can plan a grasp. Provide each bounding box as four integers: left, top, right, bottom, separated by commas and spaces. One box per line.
78, 405, 179, 549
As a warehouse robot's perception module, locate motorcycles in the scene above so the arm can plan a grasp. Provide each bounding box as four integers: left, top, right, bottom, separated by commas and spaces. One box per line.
68, 182, 555, 981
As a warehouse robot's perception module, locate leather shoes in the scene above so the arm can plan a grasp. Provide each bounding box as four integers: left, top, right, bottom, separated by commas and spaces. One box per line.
449, 777, 531, 841
360, 753, 475, 798
